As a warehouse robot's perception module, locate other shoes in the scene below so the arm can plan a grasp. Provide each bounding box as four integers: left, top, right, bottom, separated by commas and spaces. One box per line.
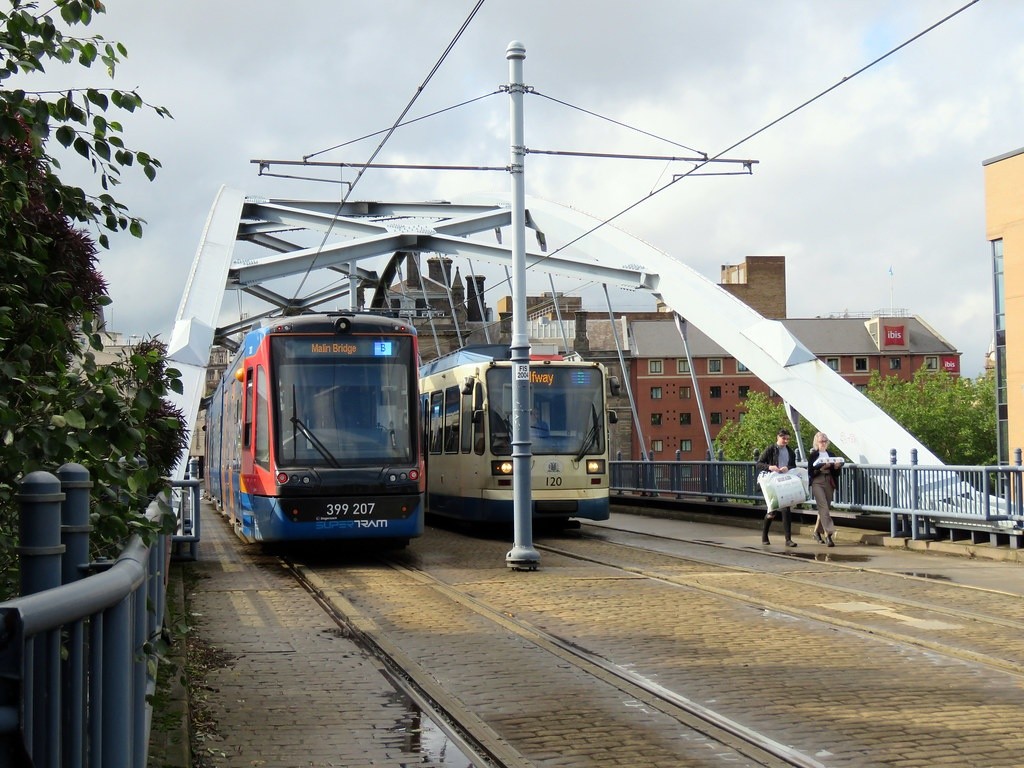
824, 534, 835, 546
762, 534, 770, 545
785, 539, 797, 547
814, 532, 824, 544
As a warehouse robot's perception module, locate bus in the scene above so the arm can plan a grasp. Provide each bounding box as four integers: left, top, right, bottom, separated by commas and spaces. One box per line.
201, 306, 427, 554
417, 340, 621, 536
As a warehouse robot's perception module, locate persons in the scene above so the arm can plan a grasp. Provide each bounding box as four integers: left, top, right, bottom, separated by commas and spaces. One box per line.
756, 429, 798, 547
529, 409, 550, 438
808, 432, 844, 547
